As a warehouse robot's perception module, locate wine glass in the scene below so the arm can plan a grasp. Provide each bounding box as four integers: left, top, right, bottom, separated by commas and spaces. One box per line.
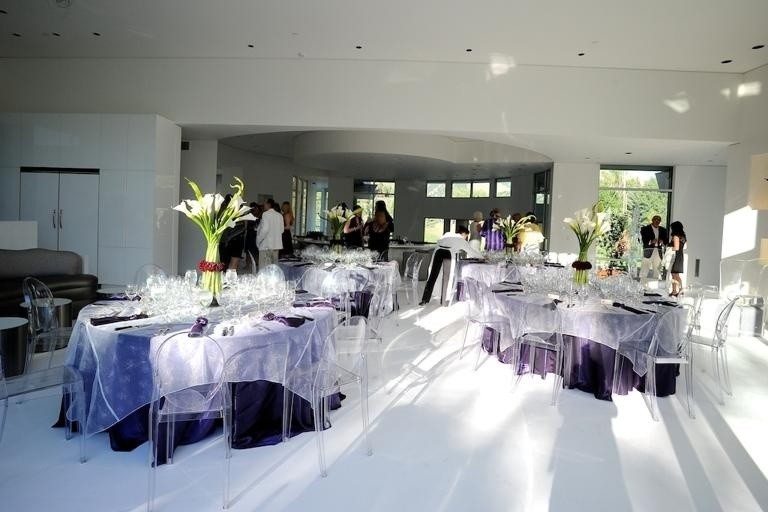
293, 243, 378, 267
517, 265, 642, 310
128, 266, 296, 327
481, 248, 542, 267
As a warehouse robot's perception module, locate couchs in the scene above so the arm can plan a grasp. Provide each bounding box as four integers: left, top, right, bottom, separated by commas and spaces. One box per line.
0, 244, 103, 326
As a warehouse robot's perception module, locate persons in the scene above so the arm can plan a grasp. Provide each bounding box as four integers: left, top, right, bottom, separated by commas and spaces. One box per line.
639, 215, 668, 283
666, 221, 687, 297
419, 224, 492, 306
453, 209, 548, 255
217, 194, 294, 274
338, 200, 395, 260
601, 221, 631, 276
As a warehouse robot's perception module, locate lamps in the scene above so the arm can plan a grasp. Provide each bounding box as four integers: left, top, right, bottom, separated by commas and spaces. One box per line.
0, 220, 42, 253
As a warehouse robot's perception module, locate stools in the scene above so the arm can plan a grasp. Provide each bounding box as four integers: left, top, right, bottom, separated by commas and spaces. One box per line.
0, 313, 33, 380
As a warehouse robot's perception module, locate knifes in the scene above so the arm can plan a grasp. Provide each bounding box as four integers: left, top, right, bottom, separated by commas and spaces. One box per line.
228, 325, 234, 340
114, 324, 152, 333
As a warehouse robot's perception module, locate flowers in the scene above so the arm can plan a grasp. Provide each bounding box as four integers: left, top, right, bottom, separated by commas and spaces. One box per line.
560, 206, 612, 283
490, 213, 537, 248
173, 173, 258, 291
317, 204, 364, 249
